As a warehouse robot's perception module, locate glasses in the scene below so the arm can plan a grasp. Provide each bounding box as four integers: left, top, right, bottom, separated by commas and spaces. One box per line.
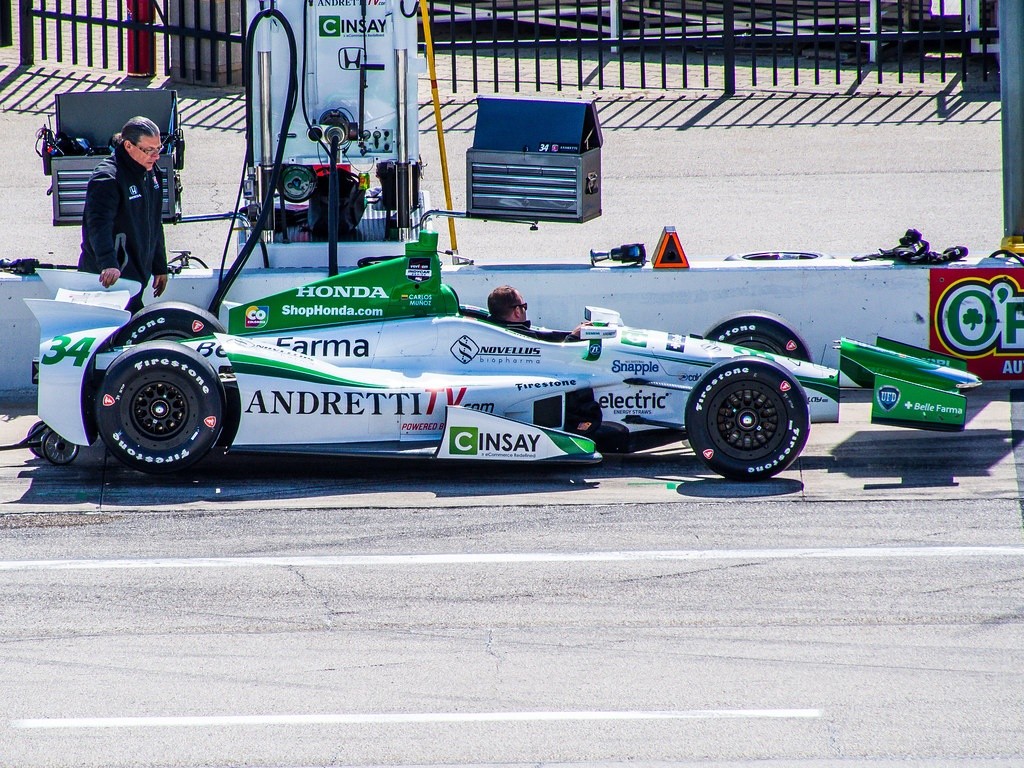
130, 141, 163, 154
510, 303, 528, 310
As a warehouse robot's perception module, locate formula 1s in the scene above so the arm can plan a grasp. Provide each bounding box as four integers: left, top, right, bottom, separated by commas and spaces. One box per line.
23, 228, 985, 487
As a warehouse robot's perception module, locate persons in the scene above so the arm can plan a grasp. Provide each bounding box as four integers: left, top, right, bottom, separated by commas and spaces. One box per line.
78, 116, 168, 318
487, 285, 593, 344
